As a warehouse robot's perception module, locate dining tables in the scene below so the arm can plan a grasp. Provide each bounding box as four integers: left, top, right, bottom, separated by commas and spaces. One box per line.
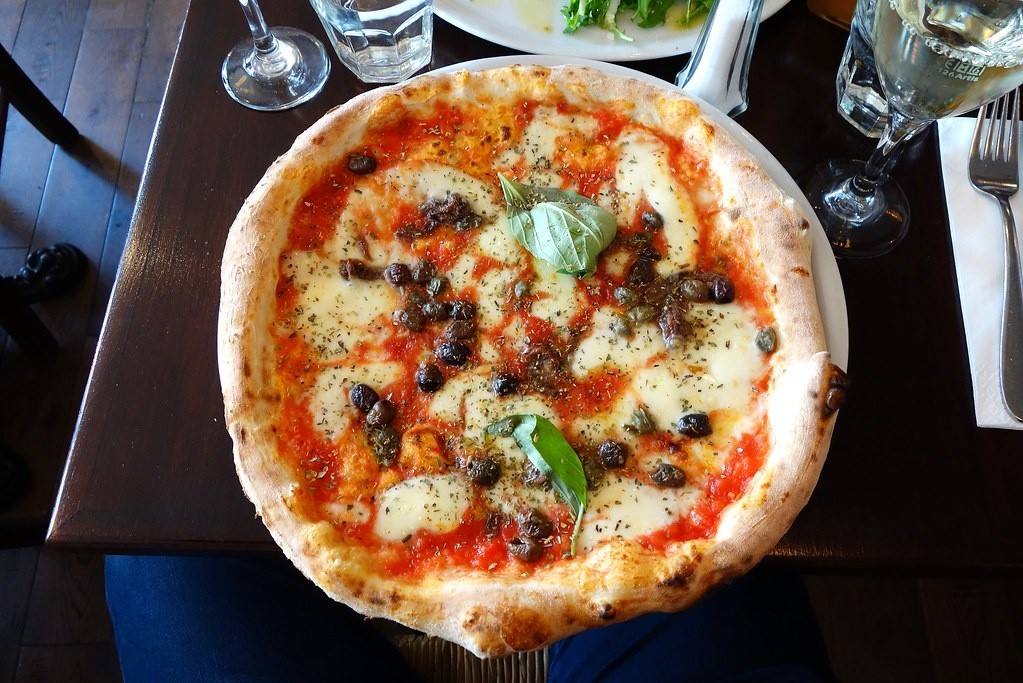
43, 0, 1023, 578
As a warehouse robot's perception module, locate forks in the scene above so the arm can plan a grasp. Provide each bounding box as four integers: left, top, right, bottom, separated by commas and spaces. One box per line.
967, 85, 1023, 422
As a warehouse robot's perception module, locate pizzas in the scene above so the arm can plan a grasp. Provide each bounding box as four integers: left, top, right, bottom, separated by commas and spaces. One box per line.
215, 67, 851, 660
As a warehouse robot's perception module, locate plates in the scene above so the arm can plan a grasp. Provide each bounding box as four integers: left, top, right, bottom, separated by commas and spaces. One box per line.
398, 54, 849, 373
433, 0, 791, 62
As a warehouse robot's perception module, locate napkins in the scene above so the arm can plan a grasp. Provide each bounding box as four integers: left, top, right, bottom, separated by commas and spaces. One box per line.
936, 116, 1023, 430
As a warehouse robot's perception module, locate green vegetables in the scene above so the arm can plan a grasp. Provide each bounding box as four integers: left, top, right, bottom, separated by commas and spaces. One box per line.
561, 0, 717, 42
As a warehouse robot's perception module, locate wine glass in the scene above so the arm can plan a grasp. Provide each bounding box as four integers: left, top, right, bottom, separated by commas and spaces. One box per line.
796, 0, 1023, 261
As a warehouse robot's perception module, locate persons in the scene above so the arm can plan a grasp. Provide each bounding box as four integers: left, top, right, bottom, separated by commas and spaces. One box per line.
100, 553, 838, 683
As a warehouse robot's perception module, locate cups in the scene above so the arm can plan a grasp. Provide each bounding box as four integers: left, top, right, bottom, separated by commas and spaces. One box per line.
309, 0, 433, 84
835, 0, 889, 138
220, 0, 331, 112
